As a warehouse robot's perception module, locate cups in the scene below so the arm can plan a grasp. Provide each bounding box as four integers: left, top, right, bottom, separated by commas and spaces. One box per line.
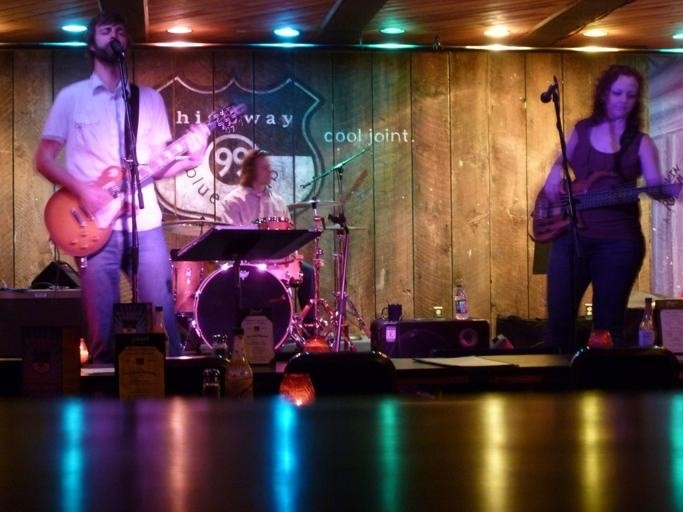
380, 304, 404, 320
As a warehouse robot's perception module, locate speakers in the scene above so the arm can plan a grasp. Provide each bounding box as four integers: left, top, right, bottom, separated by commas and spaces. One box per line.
0, 290, 85, 357
32, 261, 81, 290
372, 318, 490, 358
621, 307, 655, 350
495, 317, 590, 350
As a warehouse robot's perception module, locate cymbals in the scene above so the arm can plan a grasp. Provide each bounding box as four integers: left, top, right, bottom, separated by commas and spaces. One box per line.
163, 220, 237, 236
299, 142, 370, 189
287, 199, 337, 208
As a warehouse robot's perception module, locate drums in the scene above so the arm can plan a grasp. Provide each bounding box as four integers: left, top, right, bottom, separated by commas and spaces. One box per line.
257, 217, 297, 264
169, 248, 220, 318
193, 263, 296, 353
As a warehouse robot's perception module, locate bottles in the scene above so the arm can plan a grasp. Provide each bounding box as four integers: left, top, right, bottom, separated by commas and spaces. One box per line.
639, 296, 656, 349
196, 327, 254, 399
453, 283, 468, 321
151, 305, 170, 363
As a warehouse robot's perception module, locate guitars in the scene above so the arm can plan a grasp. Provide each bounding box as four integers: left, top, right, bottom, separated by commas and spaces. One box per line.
531, 164, 682, 241
44, 102, 254, 257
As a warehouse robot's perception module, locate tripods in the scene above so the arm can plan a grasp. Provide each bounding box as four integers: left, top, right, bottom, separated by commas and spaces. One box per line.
281, 145, 371, 353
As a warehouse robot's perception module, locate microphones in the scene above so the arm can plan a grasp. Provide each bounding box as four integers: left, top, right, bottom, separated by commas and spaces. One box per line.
109, 39, 127, 55
541, 84, 557, 104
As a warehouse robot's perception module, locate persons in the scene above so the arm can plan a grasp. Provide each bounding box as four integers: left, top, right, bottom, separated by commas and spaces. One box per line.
220, 147, 319, 343
35, 10, 208, 365
534, 65, 683, 355
